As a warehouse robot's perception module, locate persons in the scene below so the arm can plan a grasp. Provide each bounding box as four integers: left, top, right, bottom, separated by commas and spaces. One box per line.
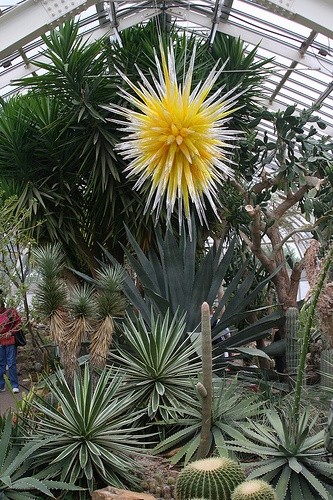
0, 289, 23, 393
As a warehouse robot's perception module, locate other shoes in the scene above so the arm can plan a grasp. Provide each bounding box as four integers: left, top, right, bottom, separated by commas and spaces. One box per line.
0, 388, 5, 391
13, 387, 19, 392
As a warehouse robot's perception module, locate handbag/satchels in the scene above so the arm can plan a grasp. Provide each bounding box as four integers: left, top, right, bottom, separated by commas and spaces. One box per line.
15, 330, 27, 347
